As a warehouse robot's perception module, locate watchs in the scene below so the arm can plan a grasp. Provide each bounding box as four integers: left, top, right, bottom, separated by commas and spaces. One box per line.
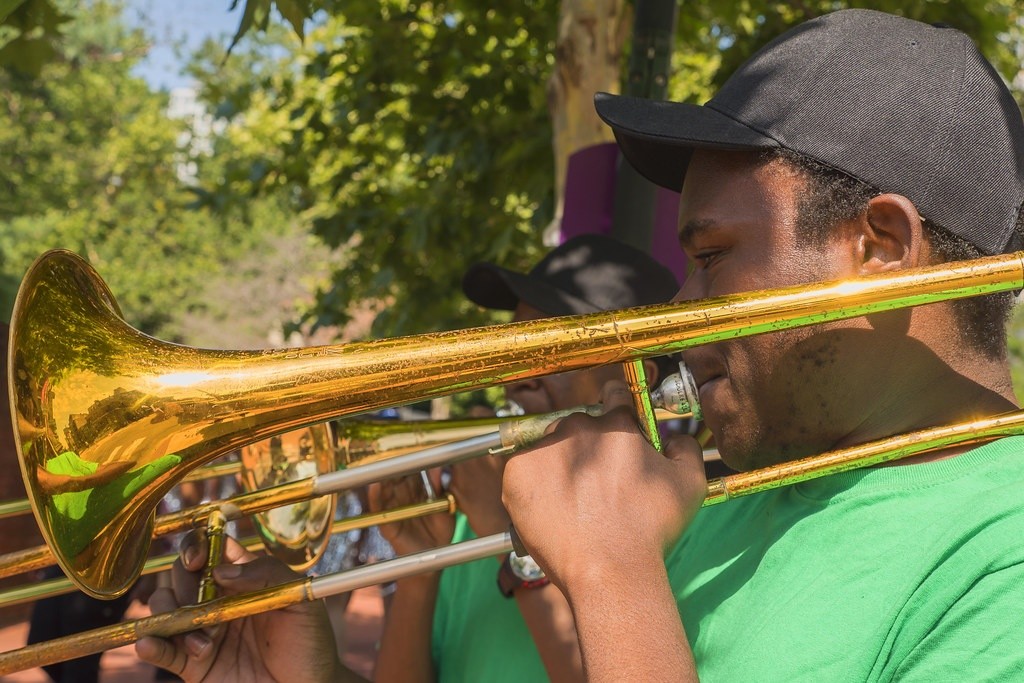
496, 548, 552, 598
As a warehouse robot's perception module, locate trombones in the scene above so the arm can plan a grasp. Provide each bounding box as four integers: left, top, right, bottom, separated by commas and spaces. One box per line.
0, 248, 1024, 679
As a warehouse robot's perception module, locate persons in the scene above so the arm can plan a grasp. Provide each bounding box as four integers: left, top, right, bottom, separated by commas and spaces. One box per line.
497, 7, 1024, 683
0, 344, 440, 683
365, 235, 681, 683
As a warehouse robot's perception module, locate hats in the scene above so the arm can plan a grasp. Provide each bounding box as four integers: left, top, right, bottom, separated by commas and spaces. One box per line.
463, 234, 680, 317
593, 8, 1024, 256
